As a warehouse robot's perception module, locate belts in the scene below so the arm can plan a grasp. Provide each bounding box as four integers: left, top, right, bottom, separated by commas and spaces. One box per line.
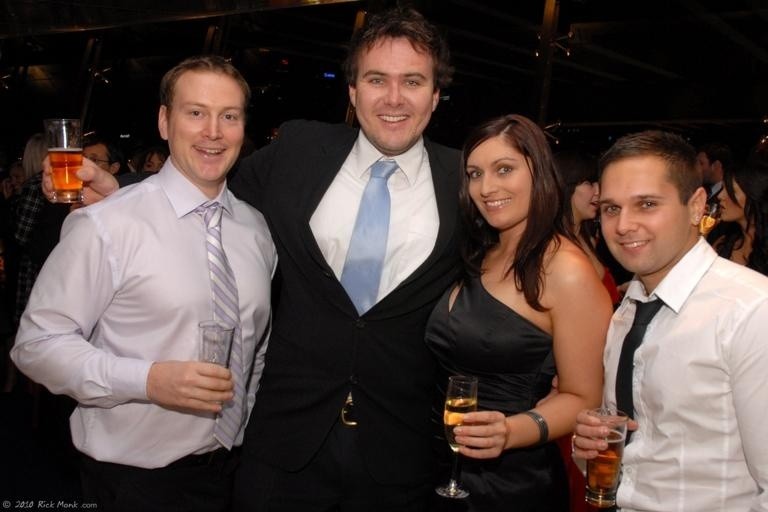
173, 448, 229, 469
339, 398, 363, 430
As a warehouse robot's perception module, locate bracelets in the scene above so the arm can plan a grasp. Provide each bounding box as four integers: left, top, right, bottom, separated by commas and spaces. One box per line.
521, 411, 548, 447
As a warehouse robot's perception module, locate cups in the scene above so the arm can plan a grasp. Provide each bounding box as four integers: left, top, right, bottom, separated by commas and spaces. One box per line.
584, 406, 628, 508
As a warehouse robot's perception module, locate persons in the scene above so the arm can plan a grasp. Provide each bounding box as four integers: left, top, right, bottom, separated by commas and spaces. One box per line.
424, 113, 614, 511
2, 55, 280, 512
538, 118, 768, 511
41, 6, 560, 511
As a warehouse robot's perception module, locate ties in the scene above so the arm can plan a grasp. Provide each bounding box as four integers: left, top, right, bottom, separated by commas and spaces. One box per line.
615, 299, 663, 444
340, 160, 399, 313
196, 205, 245, 452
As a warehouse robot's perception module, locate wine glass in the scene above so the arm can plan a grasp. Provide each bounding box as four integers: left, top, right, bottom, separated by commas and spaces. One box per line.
433, 375, 480, 501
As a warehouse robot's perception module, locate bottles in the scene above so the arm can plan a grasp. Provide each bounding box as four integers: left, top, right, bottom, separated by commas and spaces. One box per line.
39, 119, 85, 205
197, 320, 237, 406
699, 203, 721, 237
